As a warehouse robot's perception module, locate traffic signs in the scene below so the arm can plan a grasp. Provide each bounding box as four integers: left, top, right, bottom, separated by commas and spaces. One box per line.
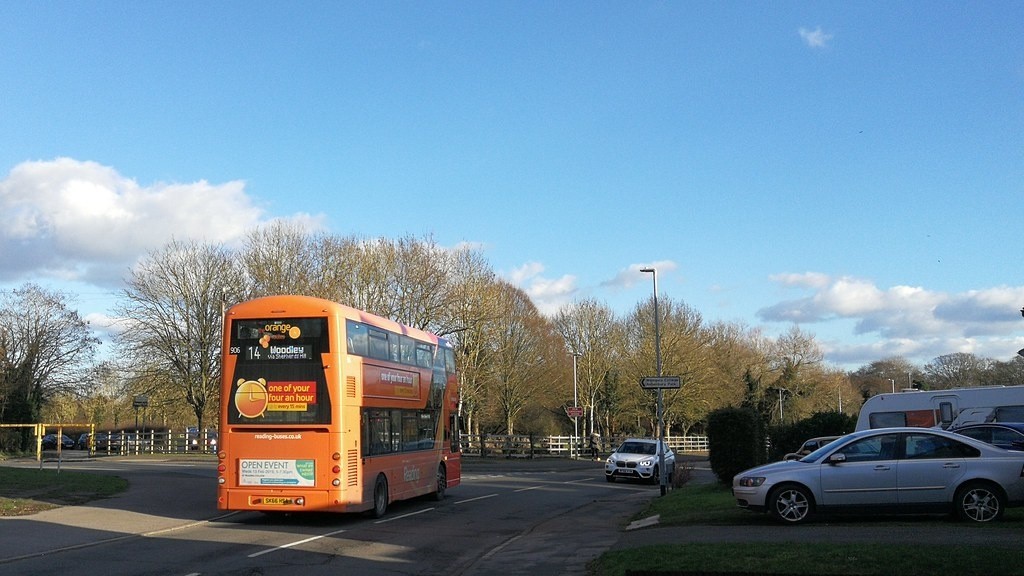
639, 375, 682, 390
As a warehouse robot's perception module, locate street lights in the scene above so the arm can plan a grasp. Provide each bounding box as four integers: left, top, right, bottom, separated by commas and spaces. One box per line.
573, 352, 582, 460
639, 267, 666, 497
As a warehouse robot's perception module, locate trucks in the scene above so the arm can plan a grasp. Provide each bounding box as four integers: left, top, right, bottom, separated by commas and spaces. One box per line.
850, 373, 1024, 433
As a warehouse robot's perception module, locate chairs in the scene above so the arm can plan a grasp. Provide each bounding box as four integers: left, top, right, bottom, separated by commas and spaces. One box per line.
878, 439, 894, 461
405, 440, 434, 450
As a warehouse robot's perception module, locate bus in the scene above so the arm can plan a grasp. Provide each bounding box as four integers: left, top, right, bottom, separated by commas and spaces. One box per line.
215, 294, 461, 521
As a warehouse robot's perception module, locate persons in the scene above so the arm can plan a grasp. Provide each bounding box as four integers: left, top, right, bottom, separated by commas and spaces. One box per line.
590, 430, 601, 461
192, 437, 198, 450
209, 439, 218, 455
500, 436, 518, 455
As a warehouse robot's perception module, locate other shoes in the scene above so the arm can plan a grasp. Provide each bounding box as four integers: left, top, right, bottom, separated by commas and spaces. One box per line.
597, 458, 600, 461
592, 458, 593, 461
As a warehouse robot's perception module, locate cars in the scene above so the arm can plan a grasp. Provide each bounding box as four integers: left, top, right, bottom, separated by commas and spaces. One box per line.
191, 430, 218, 450
732, 427, 1024, 527
915, 421, 1024, 453
782, 436, 880, 463
41, 433, 75, 451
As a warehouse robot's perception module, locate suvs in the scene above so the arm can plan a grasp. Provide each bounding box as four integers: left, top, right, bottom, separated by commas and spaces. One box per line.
110, 432, 150, 451
77, 433, 108, 451
605, 438, 675, 486
